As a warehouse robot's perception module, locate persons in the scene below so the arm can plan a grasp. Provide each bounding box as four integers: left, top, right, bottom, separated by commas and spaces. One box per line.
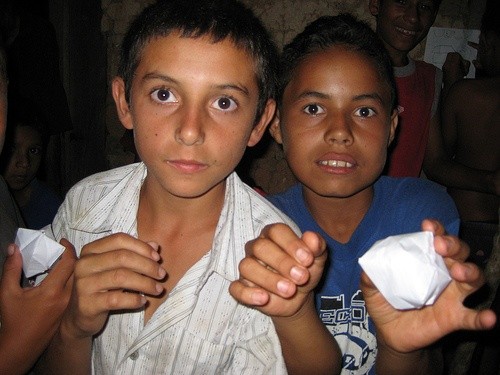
227, 16, 496, 374
36, 0, 342, 374
0, 55, 75, 374
368, 0, 499, 223
